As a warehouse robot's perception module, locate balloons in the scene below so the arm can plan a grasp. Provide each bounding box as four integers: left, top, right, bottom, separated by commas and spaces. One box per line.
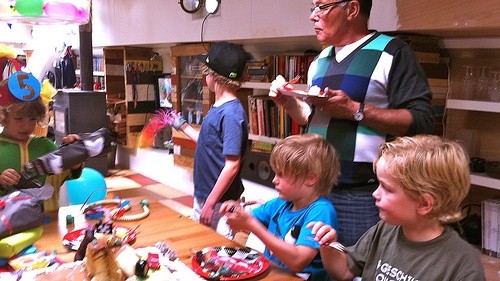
0, 0, 89, 19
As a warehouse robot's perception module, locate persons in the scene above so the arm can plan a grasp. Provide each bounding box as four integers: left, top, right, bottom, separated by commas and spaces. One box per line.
0, 72, 82, 242
164, 42, 248, 240
306, 134, 485, 281
268, 0, 435, 247
221, 133, 337, 281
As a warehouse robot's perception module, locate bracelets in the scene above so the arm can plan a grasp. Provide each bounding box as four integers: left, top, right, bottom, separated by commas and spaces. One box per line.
172, 117, 186, 131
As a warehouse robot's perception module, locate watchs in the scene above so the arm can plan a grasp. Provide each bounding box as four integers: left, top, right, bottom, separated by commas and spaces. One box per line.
352, 102, 364, 127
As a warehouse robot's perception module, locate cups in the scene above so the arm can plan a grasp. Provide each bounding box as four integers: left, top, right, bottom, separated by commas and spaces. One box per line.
481, 198, 500, 259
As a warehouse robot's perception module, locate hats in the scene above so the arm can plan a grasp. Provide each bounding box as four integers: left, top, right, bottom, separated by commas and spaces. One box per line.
196, 41, 245, 79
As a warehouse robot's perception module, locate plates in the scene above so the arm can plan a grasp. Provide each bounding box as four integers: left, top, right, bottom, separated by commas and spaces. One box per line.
63, 225, 137, 250
277, 86, 331, 99
192, 245, 270, 281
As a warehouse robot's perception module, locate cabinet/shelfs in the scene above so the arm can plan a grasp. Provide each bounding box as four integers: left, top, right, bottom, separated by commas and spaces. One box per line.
437, 38, 500, 192
241, 82, 309, 145
179, 75, 204, 104
73, 47, 124, 98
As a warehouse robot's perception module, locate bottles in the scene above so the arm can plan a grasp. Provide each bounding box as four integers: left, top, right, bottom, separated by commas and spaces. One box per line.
470, 157, 500, 179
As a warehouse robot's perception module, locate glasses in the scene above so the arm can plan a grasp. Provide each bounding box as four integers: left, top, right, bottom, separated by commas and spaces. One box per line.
310, 0, 351, 13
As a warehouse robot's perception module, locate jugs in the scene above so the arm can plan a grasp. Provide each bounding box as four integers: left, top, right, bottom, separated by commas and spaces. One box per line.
457, 203, 482, 245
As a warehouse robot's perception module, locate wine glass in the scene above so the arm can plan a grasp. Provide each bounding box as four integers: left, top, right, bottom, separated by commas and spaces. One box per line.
460, 64, 500, 103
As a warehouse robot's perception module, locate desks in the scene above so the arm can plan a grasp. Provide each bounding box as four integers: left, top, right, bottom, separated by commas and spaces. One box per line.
32, 195, 305, 281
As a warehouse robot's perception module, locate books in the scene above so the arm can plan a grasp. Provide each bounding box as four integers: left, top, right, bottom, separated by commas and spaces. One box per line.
248, 95, 308, 140
246, 55, 313, 83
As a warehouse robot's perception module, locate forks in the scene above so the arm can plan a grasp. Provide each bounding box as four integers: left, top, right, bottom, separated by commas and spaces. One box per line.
306, 234, 347, 253
282, 75, 301, 86
